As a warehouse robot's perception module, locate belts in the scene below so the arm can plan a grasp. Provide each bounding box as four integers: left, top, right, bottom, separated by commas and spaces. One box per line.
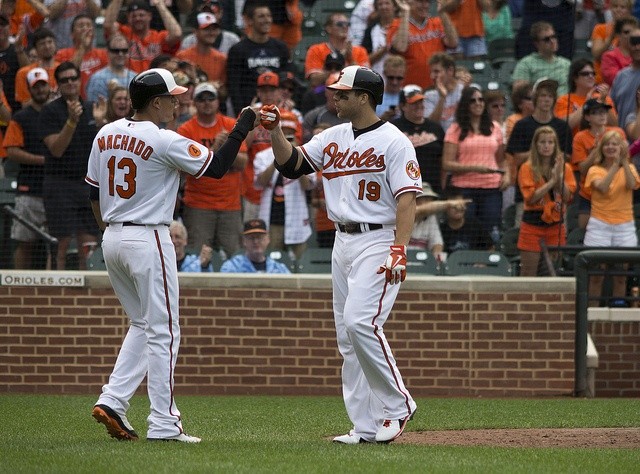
105, 222, 170, 227
334, 222, 383, 234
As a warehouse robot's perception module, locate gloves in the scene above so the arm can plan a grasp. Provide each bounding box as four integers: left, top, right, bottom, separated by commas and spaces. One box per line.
260, 104, 281, 131
381, 245, 407, 285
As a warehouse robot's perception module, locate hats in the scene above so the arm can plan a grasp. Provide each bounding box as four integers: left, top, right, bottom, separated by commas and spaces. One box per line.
583, 99, 612, 111
243, 220, 266, 235
257, 72, 281, 88
129, 68, 188, 98
326, 65, 383, 105
400, 84, 426, 104
193, 82, 218, 99
531, 77, 558, 99
27, 67, 49, 88
196, 12, 219, 29
415, 182, 438, 199
542, 201, 561, 225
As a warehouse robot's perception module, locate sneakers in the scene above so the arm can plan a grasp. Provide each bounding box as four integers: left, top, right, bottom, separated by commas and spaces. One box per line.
147, 433, 201, 443
332, 429, 372, 444
92, 404, 139, 441
376, 403, 417, 441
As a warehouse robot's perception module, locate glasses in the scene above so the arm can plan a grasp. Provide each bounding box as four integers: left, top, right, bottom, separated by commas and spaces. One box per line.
58, 76, 79, 82
108, 48, 129, 53
578, 71, 595, 76
469, 98, 485, 102
336, 21, 351, 26
542, 35, 556, 41
386, 76, 404, 80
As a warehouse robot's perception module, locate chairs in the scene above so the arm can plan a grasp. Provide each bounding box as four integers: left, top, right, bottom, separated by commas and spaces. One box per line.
86, 247, 107, 270
403, 249, 440, 276
297, 248, 332, 274
489, 38, 519, 58
567, 227, 587, 257
294, 37, 328, 73
501, 204, 518, 228
492, 58, 518, 82
190, 246, 223, 272
230, 245, 300, 273
0, 177, 19, 204
455, 56, 493, 82
566, 204, 581, 227
501, 228, 531, 260
442, 249, 513, 276
311, 0, 358, 33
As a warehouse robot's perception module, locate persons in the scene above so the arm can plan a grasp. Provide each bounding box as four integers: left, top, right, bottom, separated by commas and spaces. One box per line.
253, 112, 314, 273
252, 70, 304, 124
107, 82, 131, 120
439, 187, 495, 259
168, 221, 211, 273
553, 59, 616, 134
483, 91, 507, 143
408, 182, 472, 270
1, 1, 50, 52
227, 1, 291, 116
506, 77, 572, 240
88, 35, 139, 101
84, 68, 262, 443
39, 60, 107, 270
45, 0, 100, 52
389, 0, 458, 88
305, 13, 371, 104
177, 82, 247, 257
16, 30, 60, 108
519, 126, 576, 276
303, 74, 350, 144
262, 66, 423, 443
376, 55, 407, 117
482, 2, 512, 41
389, 84, 445, 200
610, 29, 638, 128
56, 14, 108, 98
295, 48, 358, 101
238, 70, 301, 228
514, 22, 572, 96
175, 13, 227, 114
592, 0, 631, 84
219, 220, 289, 274
4, 67, 54, 270
1, 79, 11, 173
443, 87, 506, 232
583, 131, 640, 308
439, 0, 488, 59
602, 18, 639, 84
625, 88, 640, 142
370, 1, 394, 60
180, 2, 240, 57
503, 80, 533, 225
347, 1, 374, 48
242, 1, 316, 56
1, 15, 32, 113
424, 55, 466, 129
106, 1, 183, 73
573, 97, 629, 231
